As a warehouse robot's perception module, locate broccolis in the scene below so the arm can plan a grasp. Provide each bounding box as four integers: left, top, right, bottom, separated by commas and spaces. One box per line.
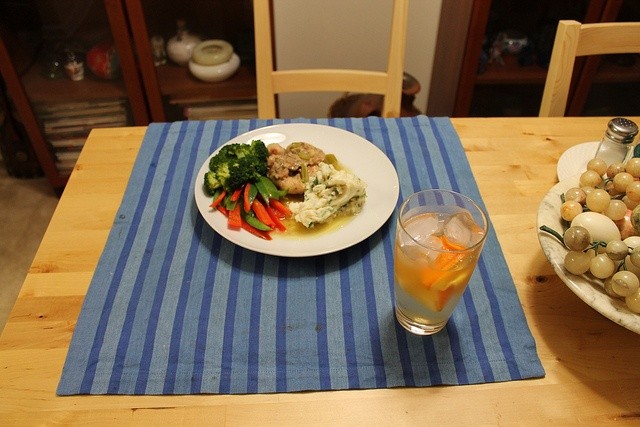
204, 138, 269, 196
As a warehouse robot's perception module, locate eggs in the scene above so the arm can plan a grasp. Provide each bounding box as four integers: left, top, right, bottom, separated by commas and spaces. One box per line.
569, 211, 621, 254
617, 236, 640, 276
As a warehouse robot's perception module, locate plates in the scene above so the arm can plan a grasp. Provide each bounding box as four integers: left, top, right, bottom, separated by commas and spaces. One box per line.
557, 141, 635, 181
537, 171, 640, 336
194, 123, 399, 259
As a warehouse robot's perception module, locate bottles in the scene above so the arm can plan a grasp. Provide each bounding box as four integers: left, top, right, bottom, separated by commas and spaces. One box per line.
594, 117, 639, 168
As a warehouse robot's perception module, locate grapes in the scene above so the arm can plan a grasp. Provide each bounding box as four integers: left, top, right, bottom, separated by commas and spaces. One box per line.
540, 150, 640, 314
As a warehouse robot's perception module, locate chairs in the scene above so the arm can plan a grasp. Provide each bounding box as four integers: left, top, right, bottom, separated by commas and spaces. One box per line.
538, 16, 639, 117
252, 2, 407, 117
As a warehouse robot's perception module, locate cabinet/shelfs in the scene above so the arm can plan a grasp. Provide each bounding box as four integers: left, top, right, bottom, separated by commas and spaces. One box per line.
0, 2, 278, 198
427, 0, 639, 118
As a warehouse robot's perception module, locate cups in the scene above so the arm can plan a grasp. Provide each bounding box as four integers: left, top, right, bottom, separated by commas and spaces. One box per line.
394, 188, 488, 336
